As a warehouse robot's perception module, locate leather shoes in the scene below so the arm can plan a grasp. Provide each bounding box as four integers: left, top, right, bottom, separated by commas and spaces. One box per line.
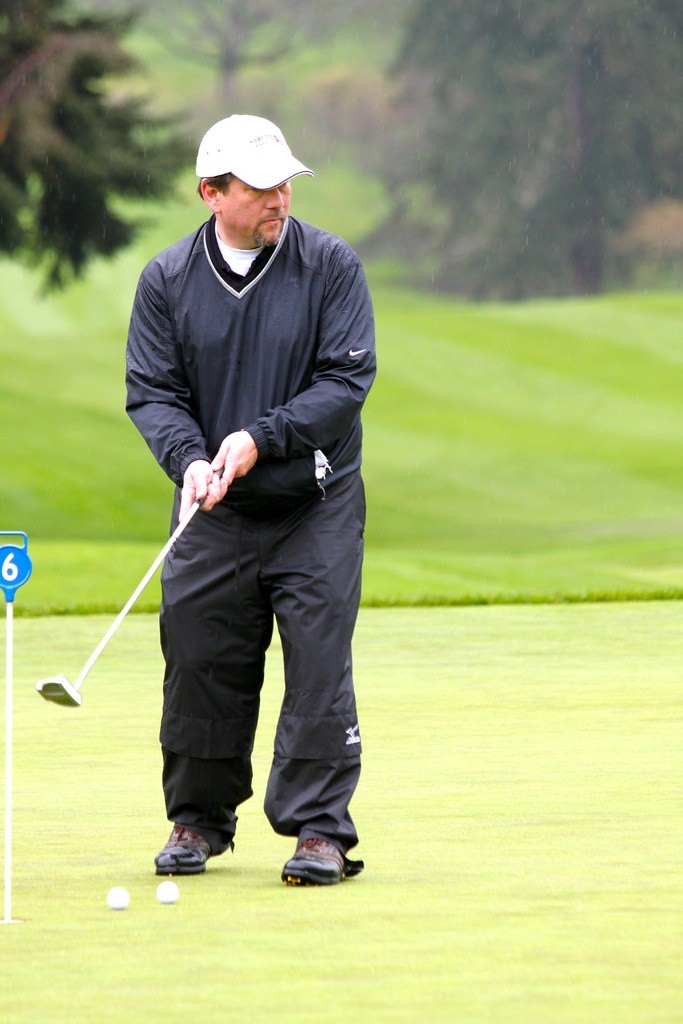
282, 837, 347, 885
154, 824, 210, 875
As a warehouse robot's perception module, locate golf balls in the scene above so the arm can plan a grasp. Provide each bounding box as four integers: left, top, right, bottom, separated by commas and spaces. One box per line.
108, 887, 129, 909
157, 881, 179, 902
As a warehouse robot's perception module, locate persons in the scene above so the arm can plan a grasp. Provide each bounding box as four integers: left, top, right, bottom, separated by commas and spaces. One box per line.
125, 115, 377, 885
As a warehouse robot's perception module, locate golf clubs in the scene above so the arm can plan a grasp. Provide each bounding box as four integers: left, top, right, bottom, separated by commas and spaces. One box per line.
39, 466, 223, 707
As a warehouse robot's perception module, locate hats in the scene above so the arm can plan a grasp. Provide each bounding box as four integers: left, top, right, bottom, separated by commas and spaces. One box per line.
196, 112, 314, 190
